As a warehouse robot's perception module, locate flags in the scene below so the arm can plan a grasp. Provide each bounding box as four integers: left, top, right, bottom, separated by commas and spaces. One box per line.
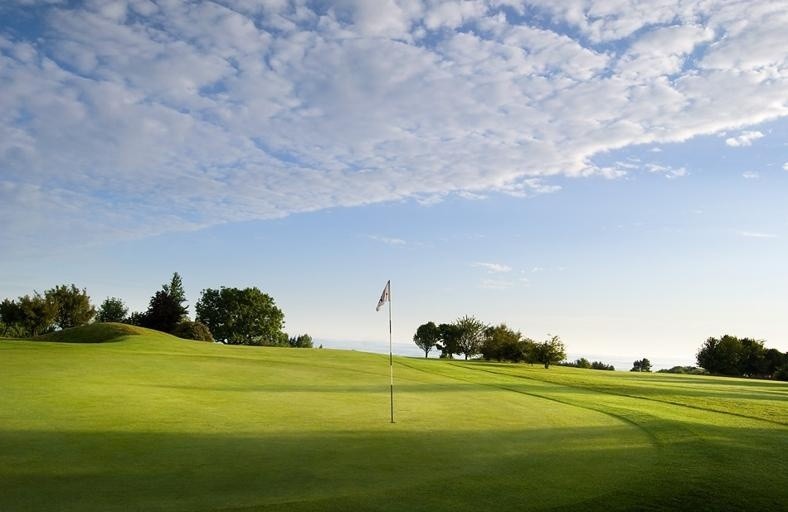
376, 284, 388, 312
